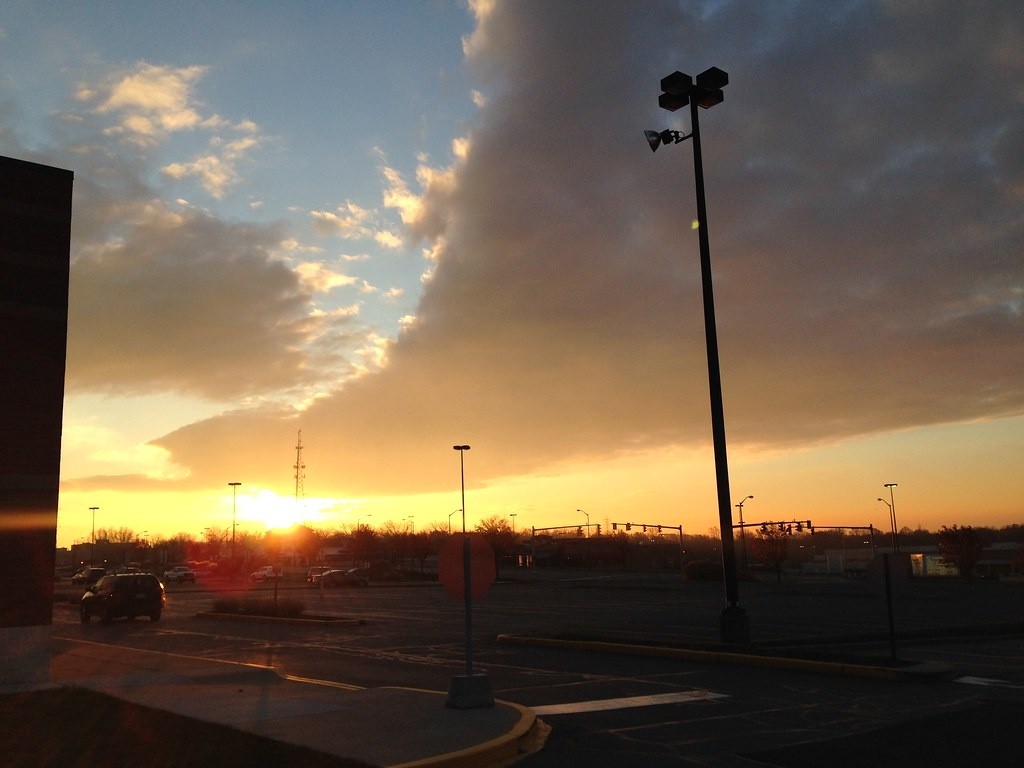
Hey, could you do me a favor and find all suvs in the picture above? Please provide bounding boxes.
[80,569,165,624]
[251,565,283,583]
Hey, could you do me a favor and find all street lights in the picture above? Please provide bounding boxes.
[576,510,589,538]
[228,483,241,580]
[510,514,517,536]
[740,496,754,566]
[453,445,470,532]
[89,507,99,568]
[449,509,463,534]
[658,67,749,646]
[878,483,900,555]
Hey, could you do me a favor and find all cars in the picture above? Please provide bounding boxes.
[306,567,369,588]
[191,568,214,579]
[120,567,142,573]
[72,567,107,586]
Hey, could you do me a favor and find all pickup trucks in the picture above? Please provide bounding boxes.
[164,567,196,585]
